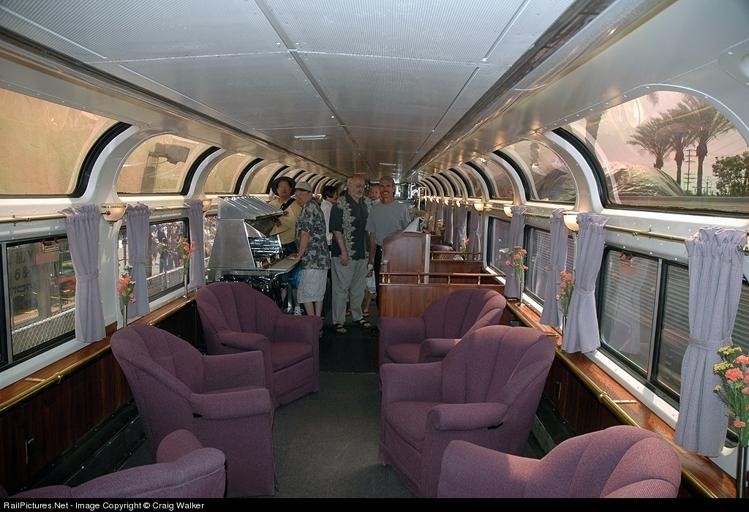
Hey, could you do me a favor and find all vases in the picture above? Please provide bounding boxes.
[561,315,568,343]
[123,304,127,325]
[734,442,745,499]
[182,272,191,298]
[519,279,525,306]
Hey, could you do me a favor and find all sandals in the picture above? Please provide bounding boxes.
[319,326,328,339]
[332,323,348,335]
[352,318,372,328]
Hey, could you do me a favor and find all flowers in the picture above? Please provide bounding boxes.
[555,272,574,322]
[510,242,534,284]
[712,338,748,446]
[177,235,195,277]
[119,269,134,307]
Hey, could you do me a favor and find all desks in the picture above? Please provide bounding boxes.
[207,249,305,312]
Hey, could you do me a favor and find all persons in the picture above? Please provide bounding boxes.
[146,214,219,276]
[267,173,412,339]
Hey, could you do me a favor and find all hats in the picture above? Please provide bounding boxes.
[294,180,312,193]
[271,176,297,197]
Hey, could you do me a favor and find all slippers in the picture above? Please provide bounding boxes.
[362,312,370,318]
[345,310,352,316]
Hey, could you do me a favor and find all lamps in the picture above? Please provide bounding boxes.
[101,203,127,223]
[503,206,514,219]
[473,202,484,215]
[199,197,213,219]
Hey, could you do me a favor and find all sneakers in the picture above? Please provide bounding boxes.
[293,309,304,316]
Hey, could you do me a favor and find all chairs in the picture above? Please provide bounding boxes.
[109,318,283,496]
[195,279,326,407]
[371,320,561,498]
[436,420,687,500]
[7,424,229,498]
[370,281,510,370]
